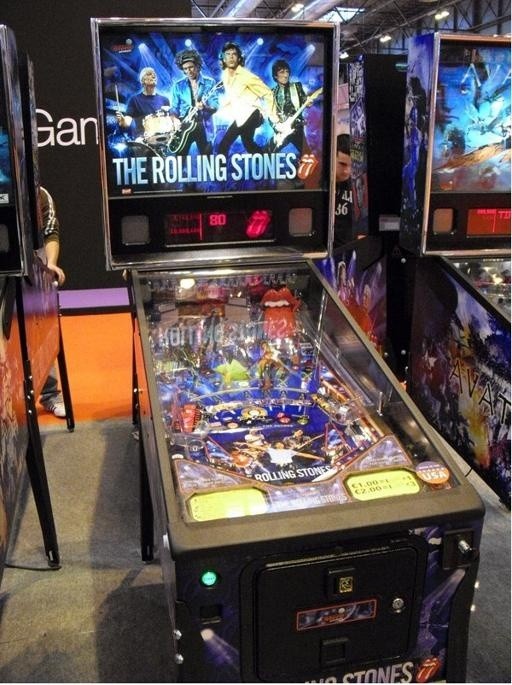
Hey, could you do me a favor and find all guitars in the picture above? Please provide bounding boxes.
[166,80,224,154]
[273,87,324,149]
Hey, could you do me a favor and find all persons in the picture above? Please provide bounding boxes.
[0,134,67,418]
[168,49,220,172]
[333,131,353,251]
[114,67,171,157]
[265,60,314,158]
[215,42,275,158]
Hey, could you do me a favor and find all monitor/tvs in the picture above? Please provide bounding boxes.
[88,18,342,270]
[417,32,511,260]
[0,23,46,280]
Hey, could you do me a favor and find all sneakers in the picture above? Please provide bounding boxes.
[40,395,66,417]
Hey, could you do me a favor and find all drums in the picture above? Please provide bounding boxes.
[143,110,181,146]
